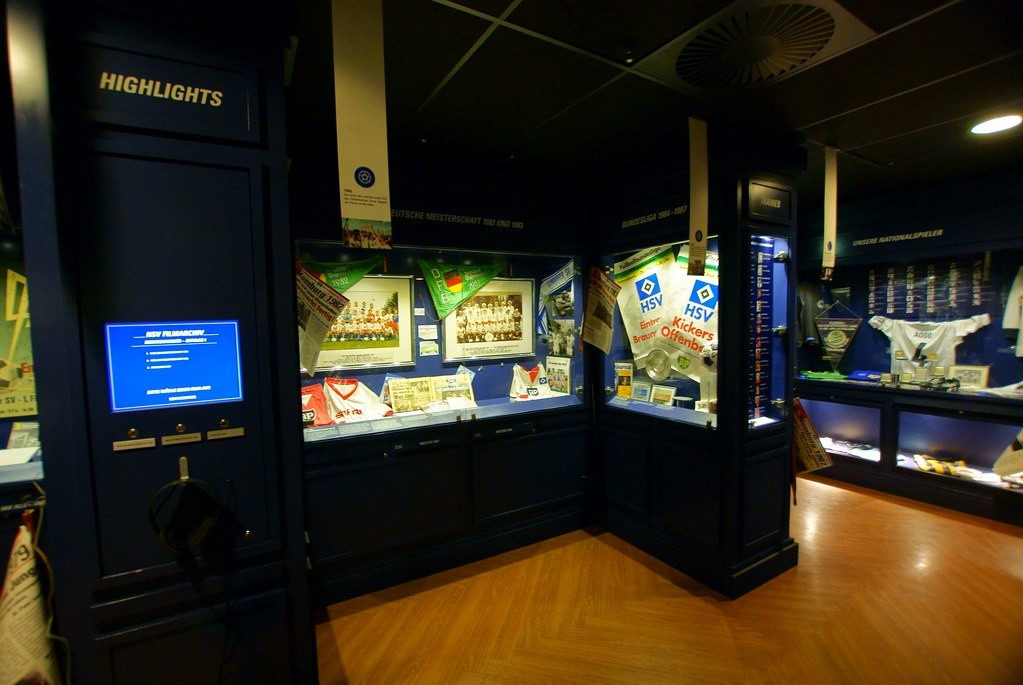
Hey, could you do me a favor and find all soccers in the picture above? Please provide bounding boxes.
[485,333,494,341]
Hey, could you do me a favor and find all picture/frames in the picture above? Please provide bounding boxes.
[442,277,536,364]
[300,274,417,374]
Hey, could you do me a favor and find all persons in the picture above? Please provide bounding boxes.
[326,301,398,341]
[396,403,422,412]
[456,301,523,342]
[619,377,627,385]
[412,381,429,392]
[955,371,978,384]
[553,290,572,315]
[547,368,566,388]
[550,327,575,356]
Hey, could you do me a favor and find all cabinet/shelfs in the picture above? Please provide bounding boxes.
[795,372,1023,526]
[310,399,798,599]
